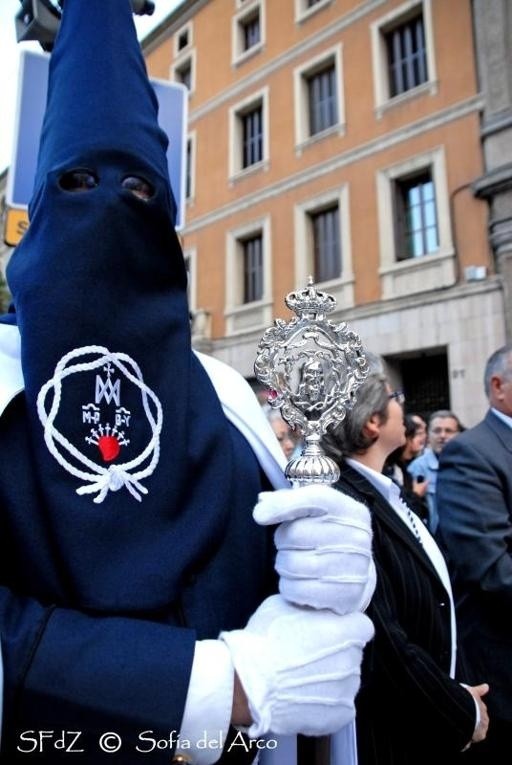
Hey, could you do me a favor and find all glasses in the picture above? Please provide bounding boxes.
[383,390,406,406]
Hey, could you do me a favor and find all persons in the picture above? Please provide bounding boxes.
[295,355,327,403]
[382,413,431,532]
[303,349,494,764]
[260,403,301,461]
[434,342,512,765]
[1,152,382,765]
[405,407,464,537]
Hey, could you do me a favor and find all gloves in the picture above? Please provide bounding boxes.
[222,592,377,737]
[252,486,375,615]
